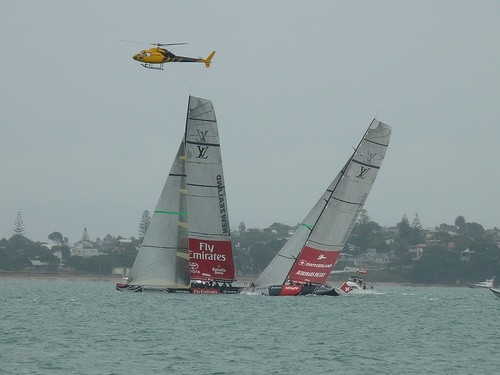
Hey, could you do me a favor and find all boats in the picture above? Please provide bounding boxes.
[466,279,494,288]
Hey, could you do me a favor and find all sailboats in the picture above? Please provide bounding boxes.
[242,119,392,296]
[118,94,245,293]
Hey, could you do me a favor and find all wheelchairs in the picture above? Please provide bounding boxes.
[312,275,369,300]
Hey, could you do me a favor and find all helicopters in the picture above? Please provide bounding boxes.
[131,43,216,71]
